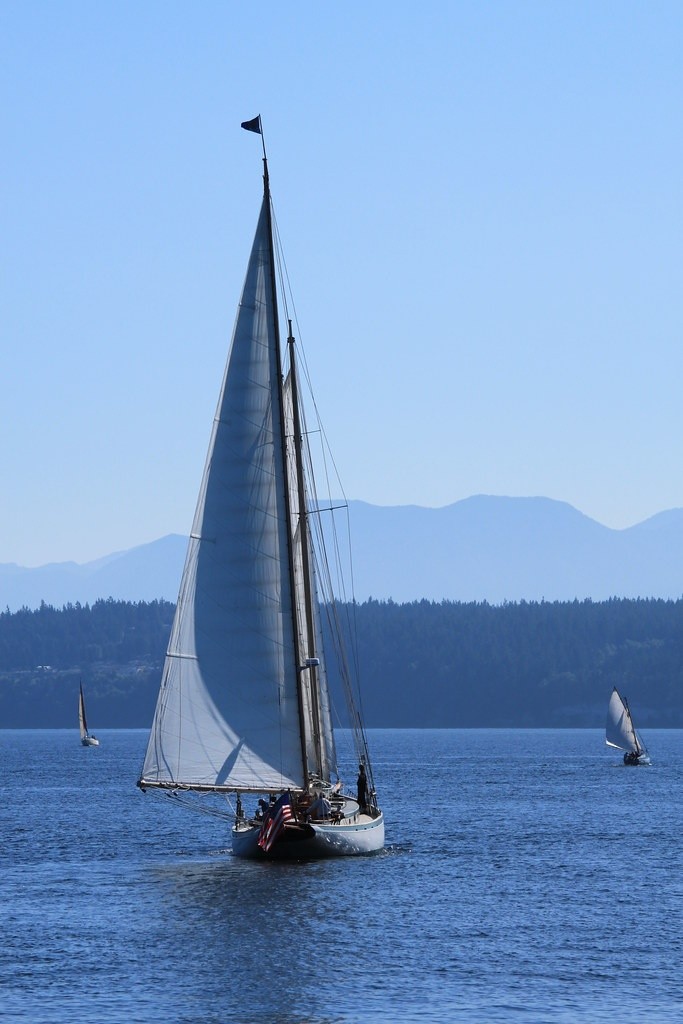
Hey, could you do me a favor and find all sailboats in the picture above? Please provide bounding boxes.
[77,678,100,746]
[135,112,386,859]
[604,685,653,765]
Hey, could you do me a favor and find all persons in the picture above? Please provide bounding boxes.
[303,790,345,822]
[259,799,269,813]
[358,764,368,814]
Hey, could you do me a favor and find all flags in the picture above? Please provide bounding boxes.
[258,790,295,851]
